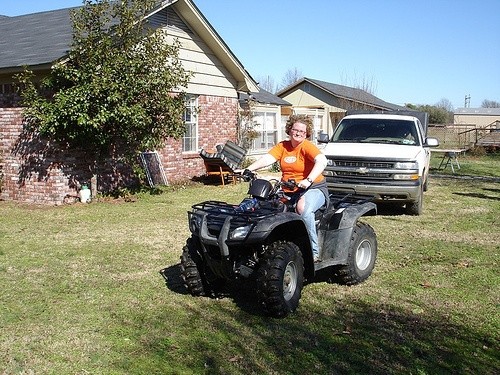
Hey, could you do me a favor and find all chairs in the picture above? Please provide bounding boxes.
[205,150,235,186]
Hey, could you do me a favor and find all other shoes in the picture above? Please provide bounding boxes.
[314,254,319,263]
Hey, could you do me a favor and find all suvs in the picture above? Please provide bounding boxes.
[317,114,440,215]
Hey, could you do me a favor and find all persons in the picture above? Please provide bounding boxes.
[234,114,330,266]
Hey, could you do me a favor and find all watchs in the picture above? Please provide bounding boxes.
[305,178,313,184]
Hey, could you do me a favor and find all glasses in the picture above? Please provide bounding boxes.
[291,129,307,135]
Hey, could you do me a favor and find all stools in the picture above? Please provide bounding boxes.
[227,172,241,184]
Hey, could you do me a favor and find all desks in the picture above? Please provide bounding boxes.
[430,149,466,173]
